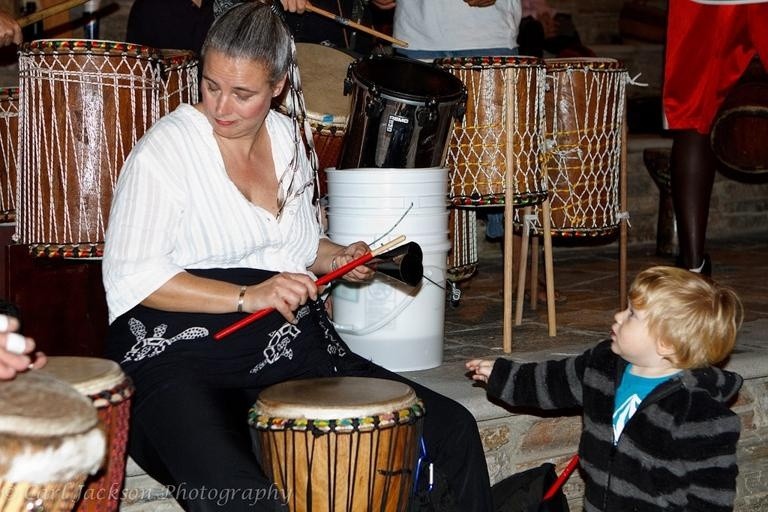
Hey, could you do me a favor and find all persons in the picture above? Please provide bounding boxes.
[465,265,744,512]
[129,0,214,105]
[376,0,523,244]
[0,312,48,380]
[663,0,768,282]
[275,0,387,67]
[0,11,24,48]
[102,3,493,512]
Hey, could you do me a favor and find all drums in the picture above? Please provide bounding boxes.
[709,56,765,183]
[29,357,135,511]
[501,58,629,245]
[0,38,199,259]
[0,369,107,511]
[337,53,468,169]
[248,376,426,512]
[295,42,358,198]
[433,55,547,212]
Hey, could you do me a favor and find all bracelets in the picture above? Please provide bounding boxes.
[238,284,247,314]
[330,257,339,273]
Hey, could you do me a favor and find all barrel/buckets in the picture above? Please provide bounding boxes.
[325,48,468,374]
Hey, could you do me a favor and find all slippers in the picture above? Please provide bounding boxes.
[501,279,568,306]
